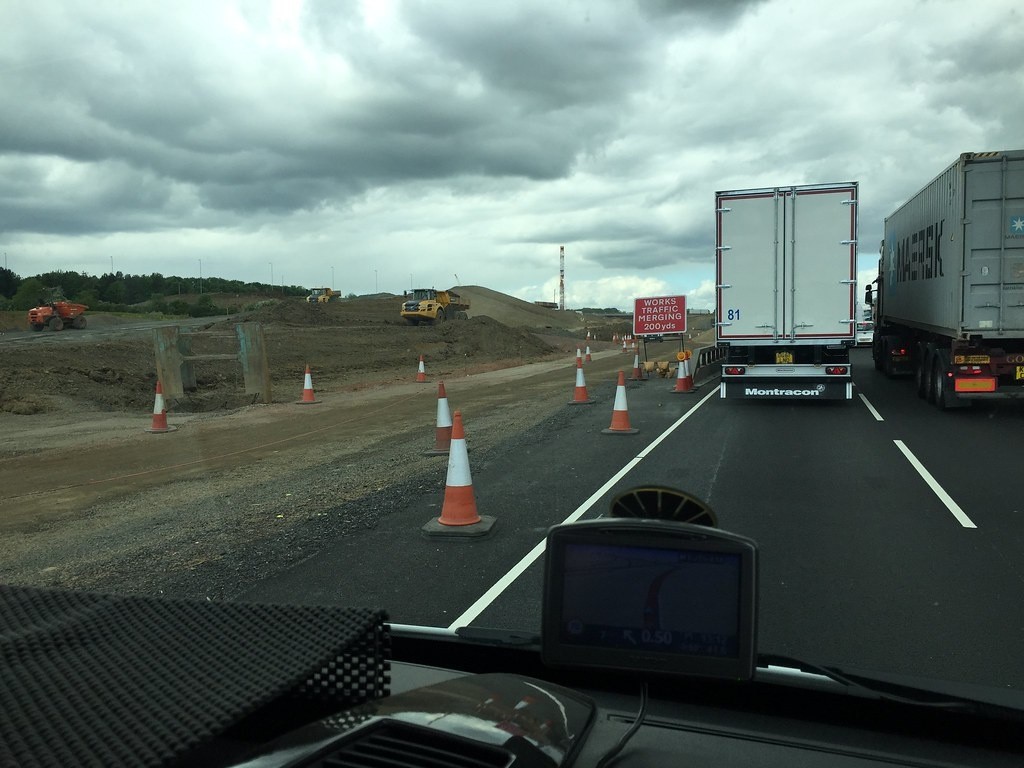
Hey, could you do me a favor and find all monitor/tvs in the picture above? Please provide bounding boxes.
[542,518,760,682]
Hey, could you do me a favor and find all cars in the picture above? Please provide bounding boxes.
[852,321,875,347]
[862,309,872,322]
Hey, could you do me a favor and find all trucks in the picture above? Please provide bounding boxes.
[400,288,469,326]
[306,288,341,303]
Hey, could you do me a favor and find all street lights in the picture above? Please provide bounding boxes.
[198,259,413,294]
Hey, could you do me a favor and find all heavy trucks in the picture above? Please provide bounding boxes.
[713,150,1024,411]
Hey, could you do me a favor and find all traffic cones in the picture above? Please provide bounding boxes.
[586,332,591,344]
[420,411,499,541]
[413,355,431,383]
[144,381,176,433]
[670,352,698,393]
[423,381,471,456]
[600,370,640,435]
[612,332,632,341]
[583,346,593,366]
[293,364,322,404]
[567,345,596,405]
[628,348,648,380]
[620,338,628,354]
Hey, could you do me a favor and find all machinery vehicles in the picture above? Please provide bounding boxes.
[28,301,89,332]
